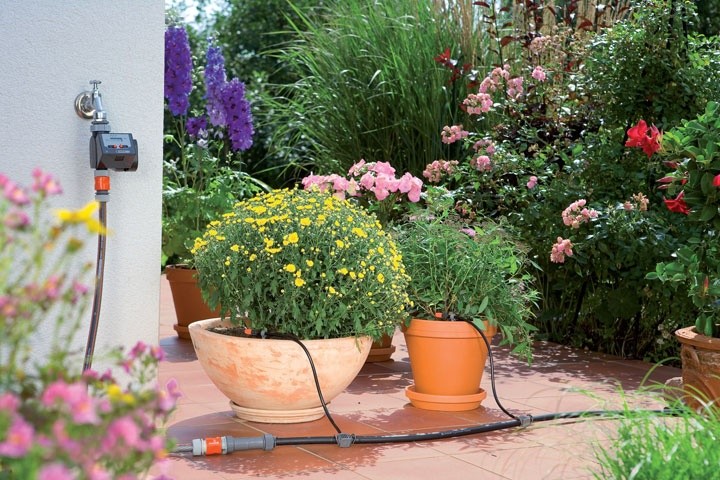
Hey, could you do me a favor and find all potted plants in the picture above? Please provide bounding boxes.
[395,220,542,411]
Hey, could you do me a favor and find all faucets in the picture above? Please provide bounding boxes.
[85,80,109,124]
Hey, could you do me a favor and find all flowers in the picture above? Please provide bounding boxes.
[189,183,414,342]
[625,99,720,337]
[161,21,274,267]
[301,157,425,233]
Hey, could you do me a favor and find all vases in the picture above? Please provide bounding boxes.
[366,326,396,362]
[181,312,371,422]
[673,323,720,410]
[165,264,231,341]
